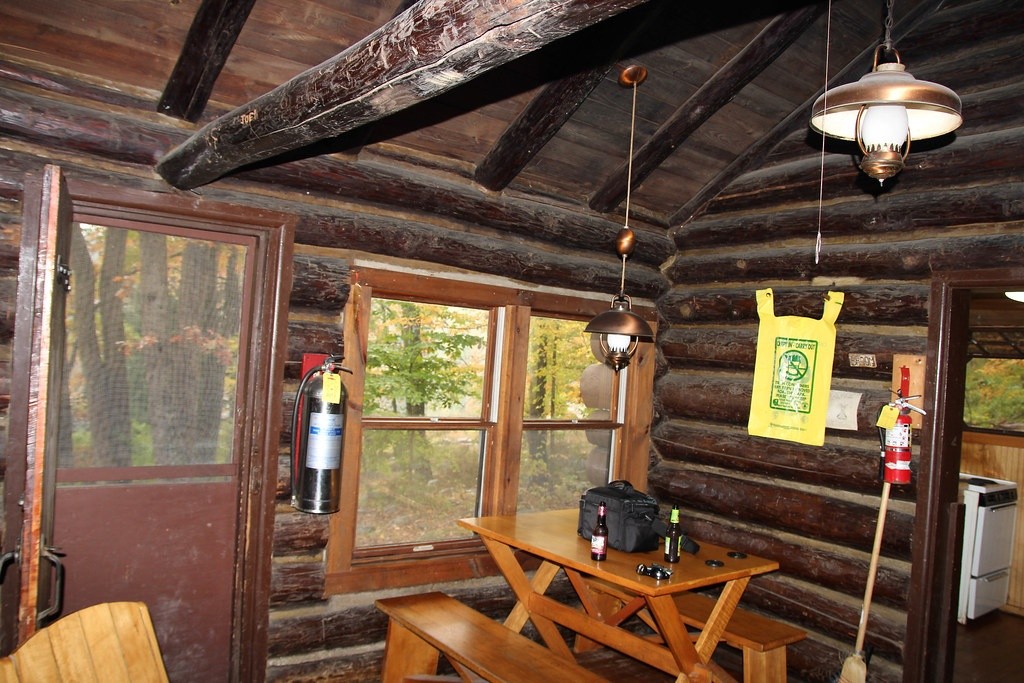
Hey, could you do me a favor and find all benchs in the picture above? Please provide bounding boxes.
[374,592,609,683]
[573,576,805,683]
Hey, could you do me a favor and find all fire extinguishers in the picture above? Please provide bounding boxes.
[876,388,927,486]
[289,354,354,514]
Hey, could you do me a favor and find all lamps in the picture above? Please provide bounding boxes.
[584,65,654,374]
[810,0,962,188]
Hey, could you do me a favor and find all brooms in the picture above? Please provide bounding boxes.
[835,482,891,683]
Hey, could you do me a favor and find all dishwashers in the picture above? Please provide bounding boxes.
[968,489,1017,620]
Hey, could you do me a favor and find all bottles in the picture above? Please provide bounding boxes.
[664,504,681,563]
[591,501,608,562]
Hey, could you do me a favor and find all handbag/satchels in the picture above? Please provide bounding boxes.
[577,479,701,554]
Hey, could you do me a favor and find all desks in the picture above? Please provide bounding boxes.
[458,508,780,683]
